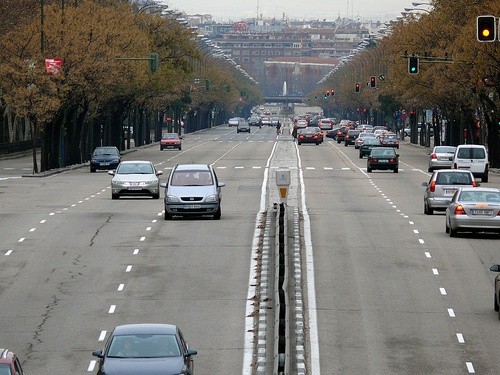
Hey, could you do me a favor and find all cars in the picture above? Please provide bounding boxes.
[443,187,500,238]
[160,133,182,151]
[422,169,481,216]
[108,160,163,201]
[0,348,23,375]
[490,265,500,320]
[93,323,197,375]
[89,146,122,173]
[449,145,489,182]
[290,112,456,173]
[160,164,225,220]
[228,105,282,134]
[123,126,133,140]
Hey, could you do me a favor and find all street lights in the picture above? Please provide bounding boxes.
[350,35,387,83]
[189,38,220,73]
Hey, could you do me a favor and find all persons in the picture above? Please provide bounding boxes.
[306,116,310,127]
[292,125,300,141]
[276,121,281,135]
[259,119,262,129]
[294,119,298,126]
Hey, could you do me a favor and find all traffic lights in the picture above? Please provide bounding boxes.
[356,84,360,93]
[408,54,419,74]
[308,89,334,102]
[371,76,376,88]
[477,16,496,42]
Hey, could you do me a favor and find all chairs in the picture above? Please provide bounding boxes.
[462,193,499,201]
[122,165,147,170]
[439,175,468,182]
[177,173,210,181]
[374,151,391,154]
